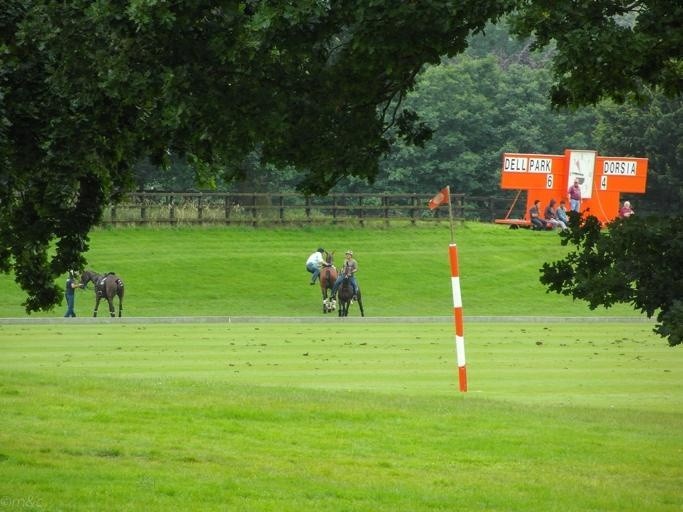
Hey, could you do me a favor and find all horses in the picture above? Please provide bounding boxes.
[78,269,127,317]
[337,264,365,317]
[318,249,338,314]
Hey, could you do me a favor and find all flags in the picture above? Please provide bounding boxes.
[425,186,448,213]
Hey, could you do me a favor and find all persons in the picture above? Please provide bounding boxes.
[304,248,330,285]
[619,200,635,219]
[62,274,84,317]
[554,200,568,225]
[528,200,547,231]
[544,199,567,232]
[566,179,582,212]
[328,250,359,303]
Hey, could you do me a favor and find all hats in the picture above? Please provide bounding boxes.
[346,250,353,256]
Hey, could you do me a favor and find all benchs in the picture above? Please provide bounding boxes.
[495,218,552,230]
